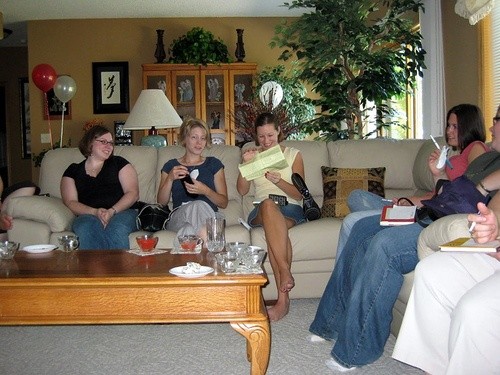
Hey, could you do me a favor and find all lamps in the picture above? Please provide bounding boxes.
[124,89,184,148]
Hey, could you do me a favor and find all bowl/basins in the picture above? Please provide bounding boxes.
[177,235,198,250]
[0,240,19,260]
[56,234,79,252]
[136,235,158,252]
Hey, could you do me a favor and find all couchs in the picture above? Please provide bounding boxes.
[2,139,473,336]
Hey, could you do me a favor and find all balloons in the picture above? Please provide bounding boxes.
[53,75,77,102]
[32,63,55,92]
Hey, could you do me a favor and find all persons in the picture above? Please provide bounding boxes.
[308,102,492,370]
[236,113,305,323]
[157,118,228,248]
[392,104,500,375]
[61,125,140,251]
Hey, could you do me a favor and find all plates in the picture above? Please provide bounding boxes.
[23,244,59,253]
[211,137,225,145]
[168,265,215,278]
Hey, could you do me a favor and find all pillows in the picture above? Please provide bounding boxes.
[320,163,389,222]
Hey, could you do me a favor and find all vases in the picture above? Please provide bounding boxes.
[155,29,164,62]
[234,29,245,62]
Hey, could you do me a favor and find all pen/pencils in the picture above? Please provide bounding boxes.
[430,134,454,169]
[468,195,492,233]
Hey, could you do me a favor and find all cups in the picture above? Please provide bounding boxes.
[216,242,266,273]
[206,213,225,252]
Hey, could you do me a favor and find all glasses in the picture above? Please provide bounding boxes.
[96,139,115,146]
[493,116,500,126]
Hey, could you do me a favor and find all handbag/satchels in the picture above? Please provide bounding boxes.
[138,201,170,232]
[292,172,321,221]
[414,175,487,228]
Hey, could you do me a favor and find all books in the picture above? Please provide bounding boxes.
[438,237,500,252]
[380,203,414,226]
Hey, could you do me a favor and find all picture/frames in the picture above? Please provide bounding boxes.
[43,75,71,119]
[90,62,131,113]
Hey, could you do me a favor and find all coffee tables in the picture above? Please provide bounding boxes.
[0,248,271,375]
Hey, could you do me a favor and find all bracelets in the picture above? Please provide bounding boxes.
[111,207,116,215]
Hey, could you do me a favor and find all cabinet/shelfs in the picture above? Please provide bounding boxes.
[141,60,258,147]
